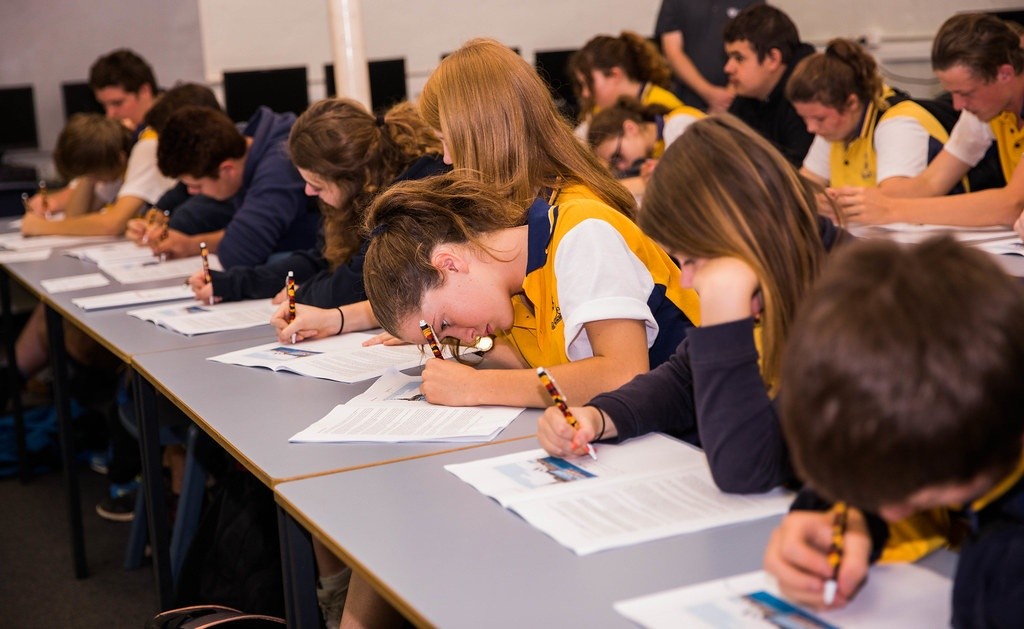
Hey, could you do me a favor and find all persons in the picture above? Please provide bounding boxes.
[0,0,1024,629]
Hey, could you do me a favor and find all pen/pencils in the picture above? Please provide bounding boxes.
[286,270,299,345]
[537,366,600,464]
[142,207,157,243]
[40,181,52,220]
[419,318,445,361]
[824,498,847,607]
[160,210,169,262]
[200,242,215,306]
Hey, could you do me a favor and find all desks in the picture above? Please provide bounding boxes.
[0,217,954,628]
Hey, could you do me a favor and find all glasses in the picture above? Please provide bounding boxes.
[608,132,626,171]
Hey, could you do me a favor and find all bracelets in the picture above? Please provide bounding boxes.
[337,308,344,334]
[594,407,606,442]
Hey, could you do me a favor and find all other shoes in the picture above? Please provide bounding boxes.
[95,452,215,522]
[315,580,349,629]
[118,401,191,438]
